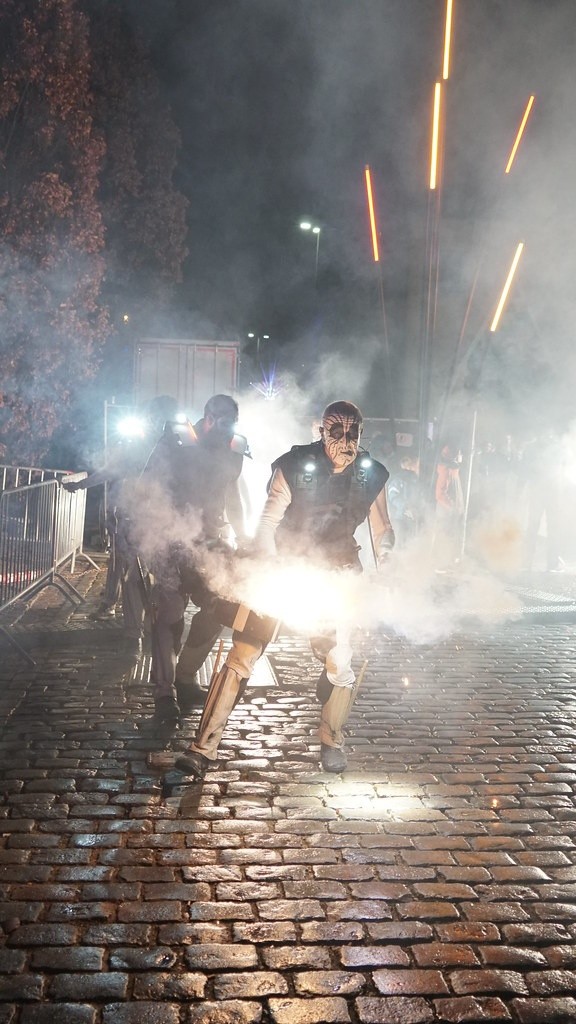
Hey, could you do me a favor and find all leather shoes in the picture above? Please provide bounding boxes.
[322,742,347,772]
[175,750,209,779]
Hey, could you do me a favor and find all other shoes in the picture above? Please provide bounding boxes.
[174,680,209,701]
[155,696,182,719]
[124,636,142,663]
[88,608,117,621]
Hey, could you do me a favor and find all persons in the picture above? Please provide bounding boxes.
[311,419,576,574]
[174,401,395,779]
[63,394,252,724]
